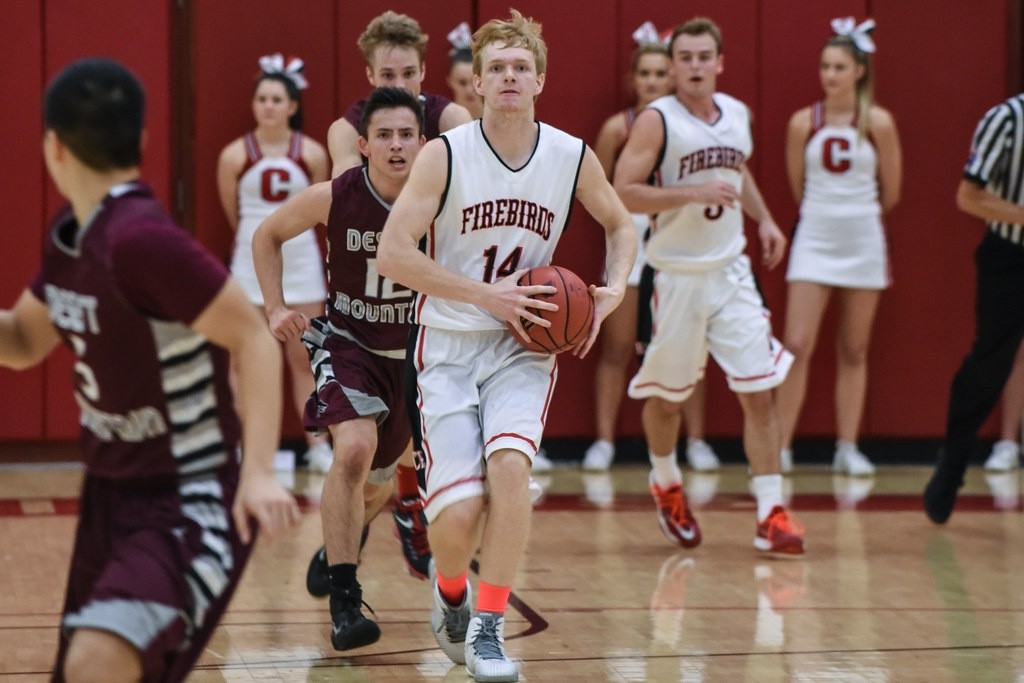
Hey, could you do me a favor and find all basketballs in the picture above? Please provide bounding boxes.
[506,266,595,355]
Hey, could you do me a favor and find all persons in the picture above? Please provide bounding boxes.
[375,8,639,683]
[445,23,483,119]
[252,87,426,652]
[922,92,1024,525]
[985,340,1024,471]
[613,16,806,560]
[327,11,474,581]
[216,53,334,473]
[0,59,302,683]
[583,21,720,471]
[773,17,901,476]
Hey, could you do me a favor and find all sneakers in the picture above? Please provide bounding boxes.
[390,500,429,582]
[646,469,703,548]
[752,506,806,558]
[983,442,1019,471]
[304,449,333,475]
[427,557,475,664]
[582,442,615,471]
[832,445,877,475]
[688,441,721,472]
[536,455,555,472]
[328,586,382,651]
[306,528,369,600]
[464,613,518,683]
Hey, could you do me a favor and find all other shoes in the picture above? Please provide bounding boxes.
[922,468,964,525]
